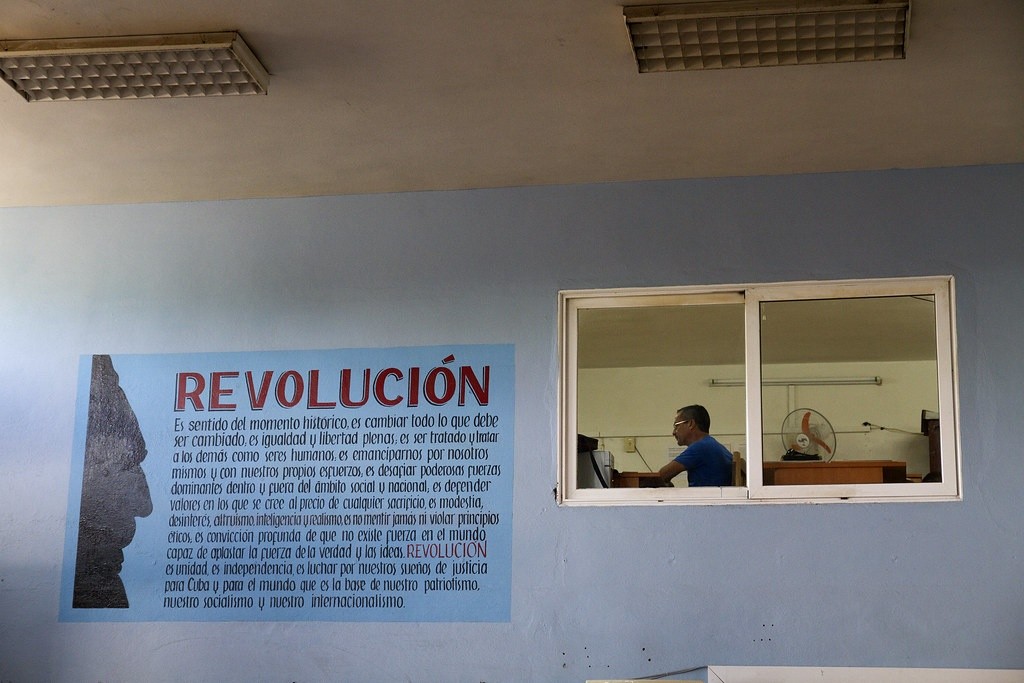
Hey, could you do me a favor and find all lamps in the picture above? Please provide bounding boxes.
[709,375,881,386]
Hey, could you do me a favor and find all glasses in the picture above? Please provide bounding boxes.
[673,419,689,427]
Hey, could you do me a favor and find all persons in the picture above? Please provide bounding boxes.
[659,405,733,487]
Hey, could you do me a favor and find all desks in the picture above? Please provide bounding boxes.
[762,460,907,485]
[613,472,662,488]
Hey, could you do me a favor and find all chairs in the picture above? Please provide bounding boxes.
[733,451,747,486]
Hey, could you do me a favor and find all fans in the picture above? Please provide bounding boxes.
[781,408,838,462]
